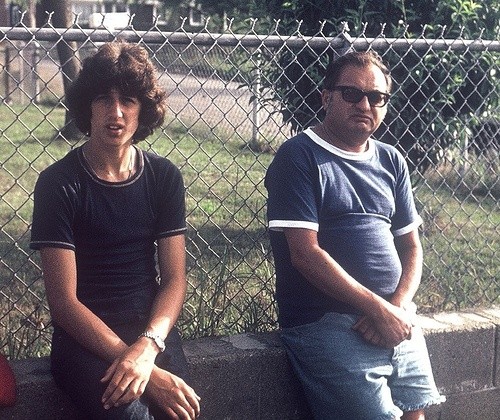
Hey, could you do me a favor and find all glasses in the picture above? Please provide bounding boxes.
[330,86,392,108]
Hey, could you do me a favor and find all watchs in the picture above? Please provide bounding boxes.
[137,332,166,352]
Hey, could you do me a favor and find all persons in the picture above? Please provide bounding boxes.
[263,50,447,420]
[31,40,203,420]
